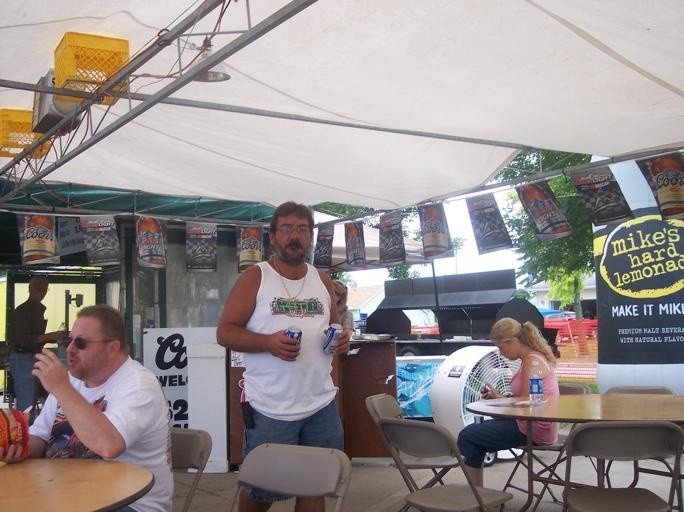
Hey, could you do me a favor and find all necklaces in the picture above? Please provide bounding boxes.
[273,255,307,299]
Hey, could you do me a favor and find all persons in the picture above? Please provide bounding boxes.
[216,203,355,511]
[330,278,353,329]
[457,318,560,488]
[0,304,172,512]
[8,276,65,426]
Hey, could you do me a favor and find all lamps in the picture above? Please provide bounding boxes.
[188,34,232,83]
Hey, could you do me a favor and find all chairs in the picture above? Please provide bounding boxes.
[602,384,682,508]
[502,384,594,512]
[170,429,212,511]
[560,420,683,511]
[366,392,465,511]
[229,443,352,512]
[377,415,515,510]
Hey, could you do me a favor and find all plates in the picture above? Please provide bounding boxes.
[351,333,392,340]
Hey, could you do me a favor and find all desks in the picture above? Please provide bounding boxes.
[464,394,683,488]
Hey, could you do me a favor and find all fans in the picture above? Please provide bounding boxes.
[430,345,523,467]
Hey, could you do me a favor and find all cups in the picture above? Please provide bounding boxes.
[359,312,367,320]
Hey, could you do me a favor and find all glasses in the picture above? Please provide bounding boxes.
[64,337,114,350]
[276,225,312,235]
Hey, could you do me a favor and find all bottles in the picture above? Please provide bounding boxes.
[55,321,67,353]
[517,184,570,240]
[343,222,366,269]
[21,215,56,263]
[238,225,263,271]
[528,360,543,405]
[420,204,448,258]
[653,153,683,218]
[416,331,422,341]
[134,215,165,268]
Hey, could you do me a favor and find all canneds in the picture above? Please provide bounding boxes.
[286,328,302,351]
[322,323,345,355]
